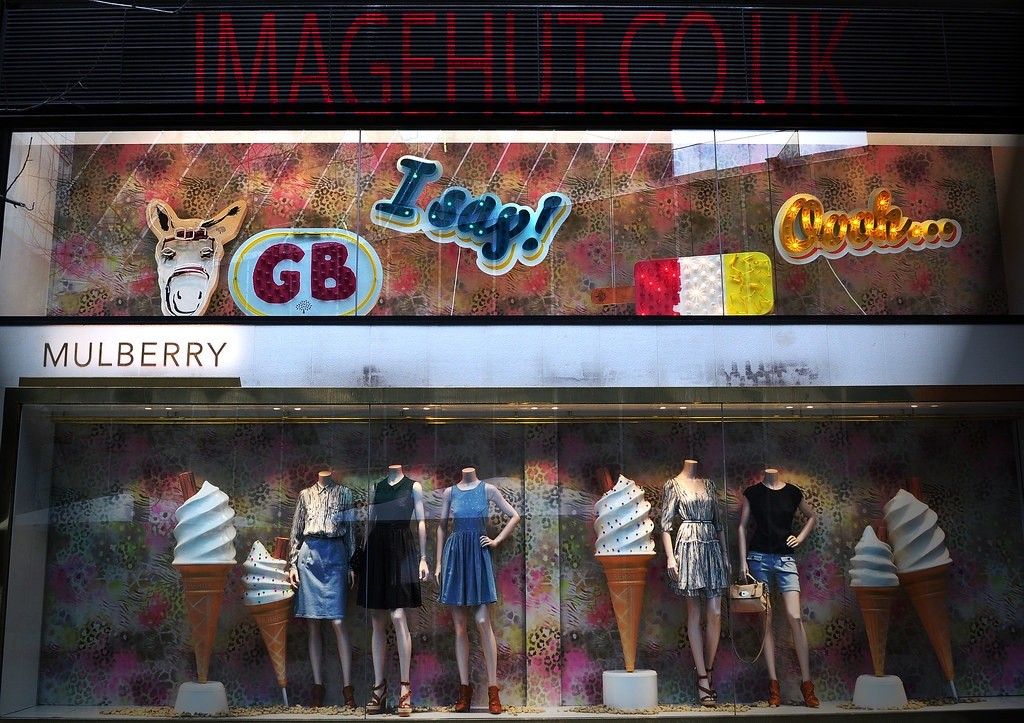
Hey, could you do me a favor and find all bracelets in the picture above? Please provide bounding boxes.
[420,556,426,559]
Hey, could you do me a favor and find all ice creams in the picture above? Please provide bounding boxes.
[173,479,296,711]
[851,487,961,700]
[592,475,656,672]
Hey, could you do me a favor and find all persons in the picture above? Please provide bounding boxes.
[737,469,820,708]
[288,471,356,712]
[661,460,732,705]
[350,464,429,716]
[432,468,520,713]
